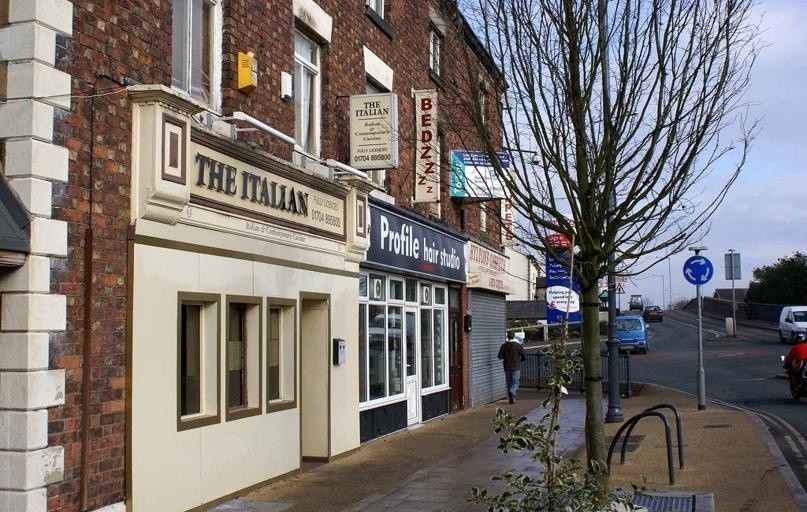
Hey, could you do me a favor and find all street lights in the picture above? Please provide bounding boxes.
[652,274,664,309]
[656,256,671,310]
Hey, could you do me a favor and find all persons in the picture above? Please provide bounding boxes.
[498,331,527,404]
[782,333,807,392]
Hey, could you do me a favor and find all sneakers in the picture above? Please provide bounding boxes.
[508,393,514,404]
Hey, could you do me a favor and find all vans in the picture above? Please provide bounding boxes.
[614,315,650,355]
[368,311,416,348]
[776,304,806,344]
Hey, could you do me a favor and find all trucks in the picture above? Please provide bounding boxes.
[627,294,643,311]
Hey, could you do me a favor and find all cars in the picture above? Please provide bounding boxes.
[642,305,663,321]
[779,340,806,400]
[505,332,523,347]
